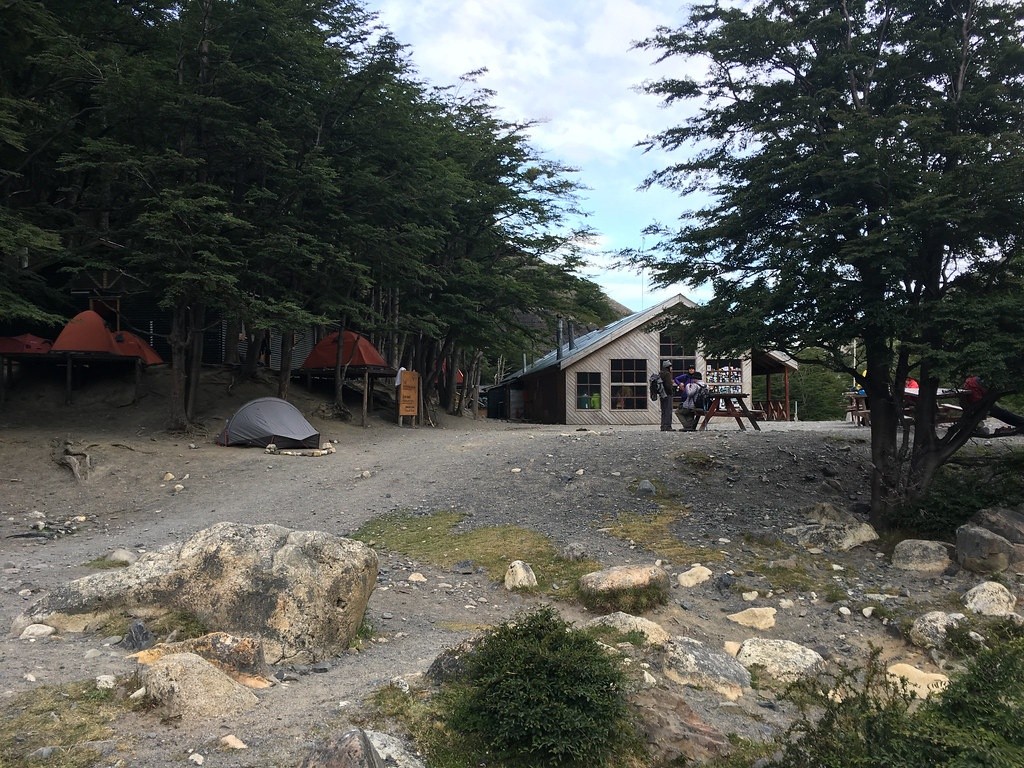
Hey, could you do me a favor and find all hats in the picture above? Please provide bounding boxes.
[690,372,702,380]
[688,364,695,369]
[663,360,672,368]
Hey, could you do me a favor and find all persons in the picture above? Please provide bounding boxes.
[856,369,871,426]
[590,390,601,409]
[905,378,919,388]
[959,375,988,420]
[659,360,707,431]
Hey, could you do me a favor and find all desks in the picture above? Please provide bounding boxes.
[847,393,870,427]
[699,393,761,431]
[904,387,971,426]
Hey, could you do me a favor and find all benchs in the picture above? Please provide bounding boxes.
[845,403,988,423]
[690,409,766,419]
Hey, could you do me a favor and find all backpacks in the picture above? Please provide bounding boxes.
[693,383,711,410]
[649,371,670,394]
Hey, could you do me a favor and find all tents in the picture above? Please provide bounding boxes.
[301,330,388,369]
[0,310,163,366]
[213,397,320,449]
[456,370,463,385]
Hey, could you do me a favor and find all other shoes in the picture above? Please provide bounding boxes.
[679,427,694,432]
[663,426,675,431]
